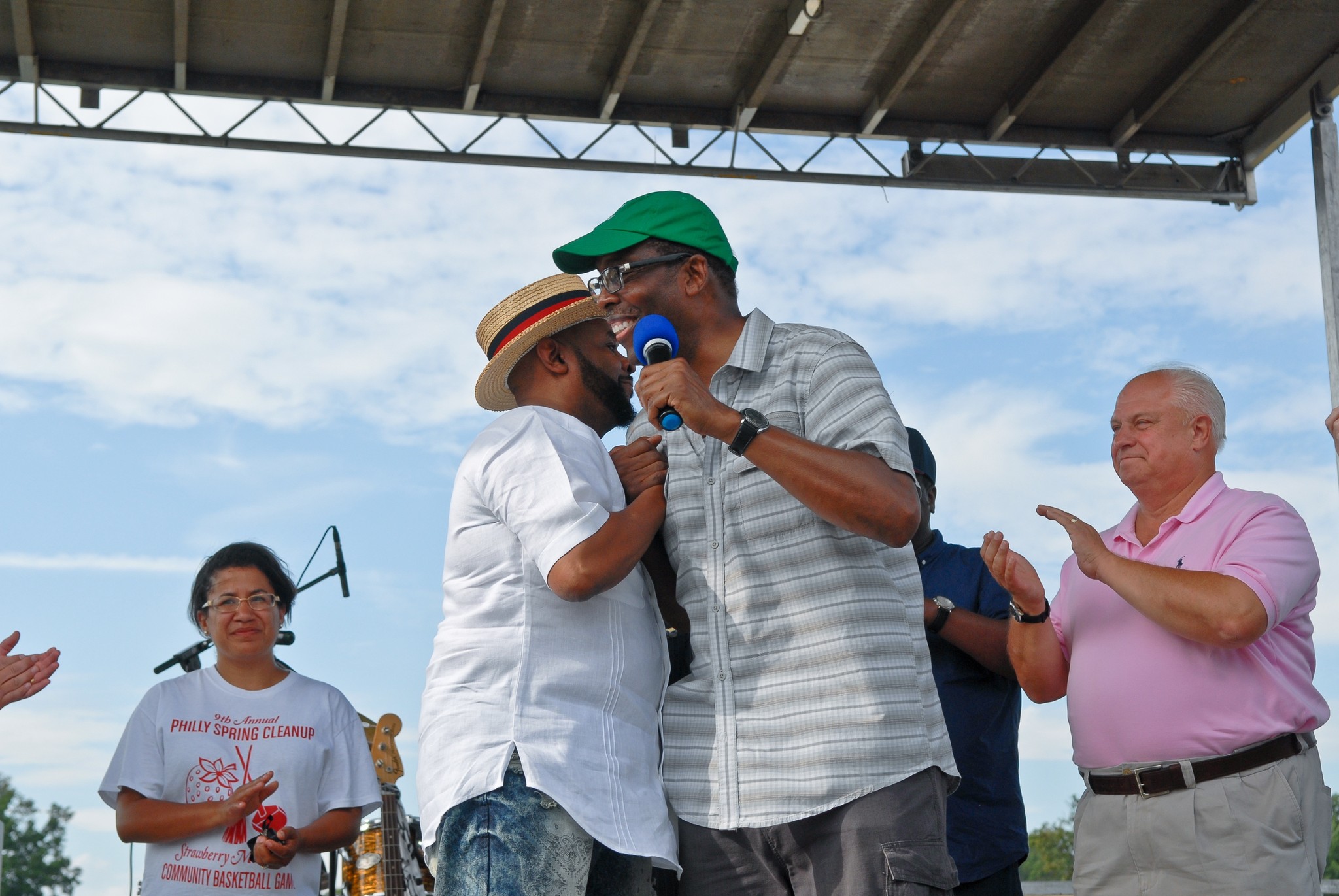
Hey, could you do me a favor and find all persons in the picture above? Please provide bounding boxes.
[905,426,1029,896]
[98,543,383,896]
[417,273,682,896]
[980,367,1332,896]
[0,631,60,710]
[552,191,963,896]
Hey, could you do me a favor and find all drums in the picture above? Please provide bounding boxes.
[348,815,425,896]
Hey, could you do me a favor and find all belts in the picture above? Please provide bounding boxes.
[1082,730,1318,796]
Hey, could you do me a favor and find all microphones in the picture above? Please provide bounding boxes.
[333,525,350,598]
[632,314,683,432]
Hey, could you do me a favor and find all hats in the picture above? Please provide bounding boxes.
[905,427,936,484]
[552,191,738,274]
[475,274,612,411]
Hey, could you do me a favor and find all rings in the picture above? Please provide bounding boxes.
[31,678,34,685]
[1071,517,1078,523]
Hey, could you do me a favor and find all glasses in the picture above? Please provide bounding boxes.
[202,592,281,613]
[587,252,715,299]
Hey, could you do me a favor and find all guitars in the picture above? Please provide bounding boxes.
[368,714,408,896]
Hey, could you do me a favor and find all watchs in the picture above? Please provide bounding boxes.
[929,596,954,634]
[1010,596,1051,624]
[728,408,769,457]
